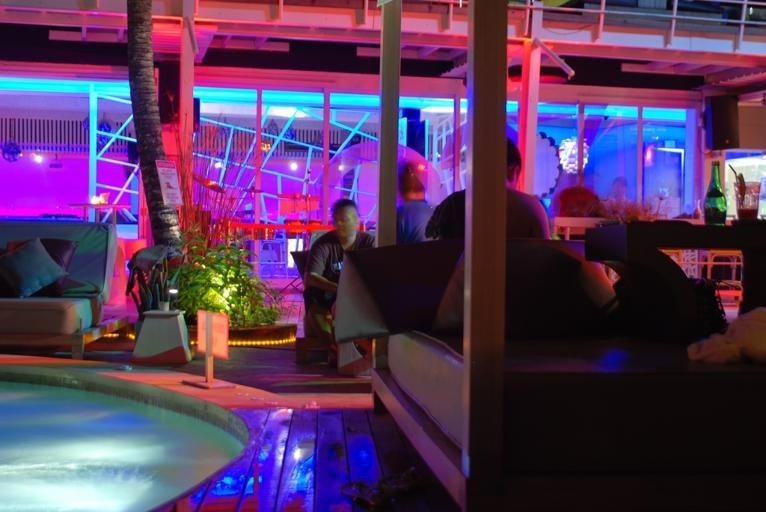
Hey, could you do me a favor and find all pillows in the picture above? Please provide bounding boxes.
[432,241,620,340]
[0,236,78,299]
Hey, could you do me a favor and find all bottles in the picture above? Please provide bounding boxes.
[703,158,727,228]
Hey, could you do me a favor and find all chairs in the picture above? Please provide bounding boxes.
[289,251,327,371]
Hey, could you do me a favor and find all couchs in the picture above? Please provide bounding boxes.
[0,220,116,334]
[331,236,766,475]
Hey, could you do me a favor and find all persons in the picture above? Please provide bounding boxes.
[601,175,635,214]
[555,173,611,216]
[425,136,549,242]
[396,161,439,246]
[302,201,377,368]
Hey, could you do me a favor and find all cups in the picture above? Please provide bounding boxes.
[733,180,762,222]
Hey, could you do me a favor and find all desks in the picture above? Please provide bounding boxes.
[584,220,766,337]
